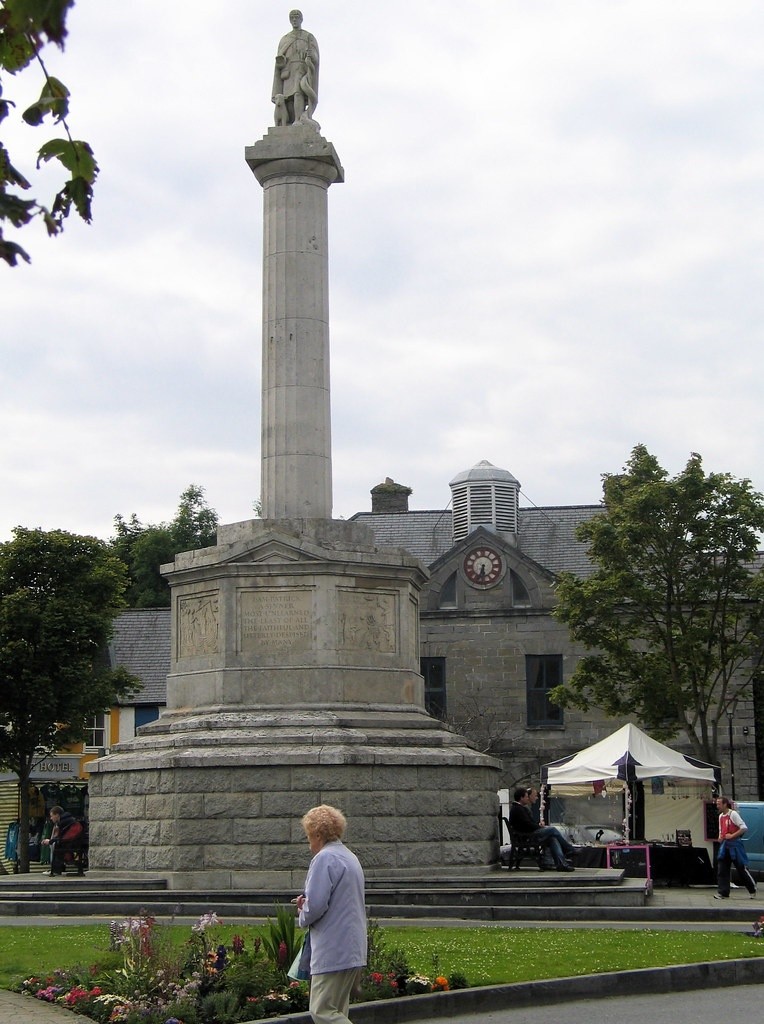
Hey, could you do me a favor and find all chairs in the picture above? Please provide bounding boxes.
[52,818,87,878]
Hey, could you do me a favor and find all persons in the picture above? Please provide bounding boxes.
[508,789,584,873]
[527,787,558,870]
[713,797,757,900]
[40,805,83,875]
[271,11,319,124]
[297,803,368,1024]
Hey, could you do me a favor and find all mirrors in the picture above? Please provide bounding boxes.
[606,845,650,878]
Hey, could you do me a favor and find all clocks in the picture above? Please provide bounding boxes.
[458,543,506,590]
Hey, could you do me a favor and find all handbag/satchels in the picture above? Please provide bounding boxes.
[286,927,312,981]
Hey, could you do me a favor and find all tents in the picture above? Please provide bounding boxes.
[539,723,715,784]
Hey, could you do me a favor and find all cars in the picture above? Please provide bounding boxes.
[550,824,626,845]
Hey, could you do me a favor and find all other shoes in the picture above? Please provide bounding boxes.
[558,863,576,872]
[41,870,59,876]
[567,848,583,859]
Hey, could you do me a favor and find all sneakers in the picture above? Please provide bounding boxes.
[749,884,757,899]
[714,893,729,900]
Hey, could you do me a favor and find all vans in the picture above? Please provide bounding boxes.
[733,801,764,883]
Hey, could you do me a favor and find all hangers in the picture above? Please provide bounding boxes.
[10,781,89,827]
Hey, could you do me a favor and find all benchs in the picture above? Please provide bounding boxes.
[501,816,548,872]
